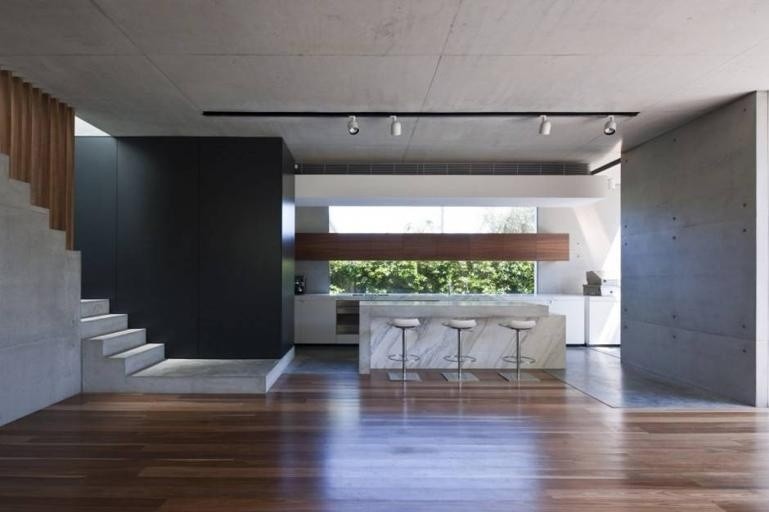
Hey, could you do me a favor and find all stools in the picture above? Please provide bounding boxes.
[388,317,541,384]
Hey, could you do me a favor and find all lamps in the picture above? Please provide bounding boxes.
[536,110,619,140]
[346,110,404,139]
